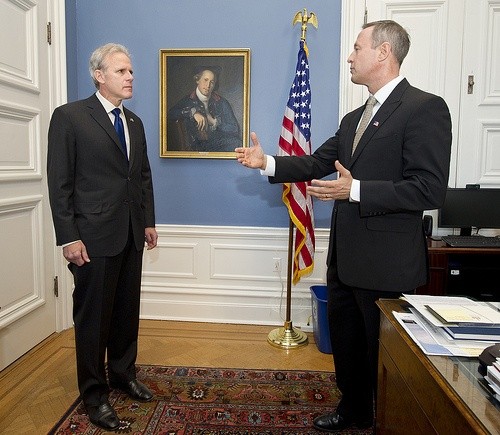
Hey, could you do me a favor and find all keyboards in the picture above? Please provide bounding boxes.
[442,235,500,247]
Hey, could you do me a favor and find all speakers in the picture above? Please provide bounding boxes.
[423,215,433,239]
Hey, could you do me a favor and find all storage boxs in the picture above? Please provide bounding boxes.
[310,286,332,353]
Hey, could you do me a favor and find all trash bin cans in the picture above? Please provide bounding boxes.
[310,285,334,354]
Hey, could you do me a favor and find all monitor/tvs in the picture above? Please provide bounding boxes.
[438,188,500,236]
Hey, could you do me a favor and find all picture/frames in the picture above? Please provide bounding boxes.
[159,48,251,158]
[272,258,281,272]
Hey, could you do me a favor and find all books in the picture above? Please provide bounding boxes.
[392,294,500,401]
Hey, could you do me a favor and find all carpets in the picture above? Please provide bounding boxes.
[47,362,374,435]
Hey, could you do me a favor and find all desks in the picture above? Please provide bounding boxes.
[427,236,500,295]
[376,296,500,435]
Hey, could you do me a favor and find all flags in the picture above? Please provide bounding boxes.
[277,40,316,285]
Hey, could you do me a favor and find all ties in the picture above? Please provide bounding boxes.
[351,95,378,158]
[111,108,128,161]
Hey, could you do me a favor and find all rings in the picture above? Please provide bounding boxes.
[326,194,327,198]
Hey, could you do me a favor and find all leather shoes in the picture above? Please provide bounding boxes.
[86,400,120,431]
[312,410,373,432]
[124,378,153,403]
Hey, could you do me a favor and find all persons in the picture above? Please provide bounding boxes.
[46,42,158,429]
[234,20,452,431]
